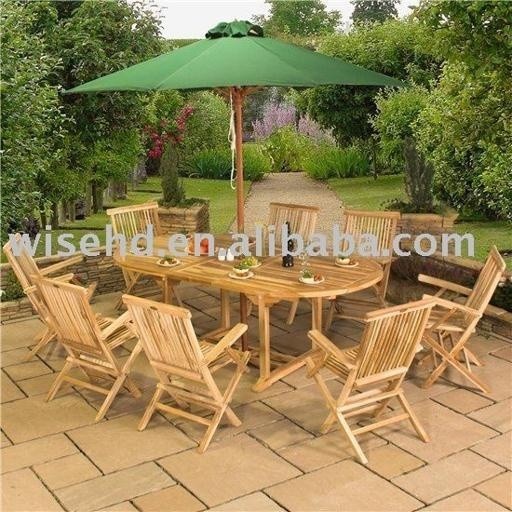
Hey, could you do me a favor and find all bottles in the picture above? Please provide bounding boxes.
[217,248,235,261]
[282,221,293,266]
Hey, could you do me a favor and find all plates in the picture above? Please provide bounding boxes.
[157,256,179,267]
[300,276,324,284]
[228,270,254,279]
[185,247,218,256]
[334,260,359,269]
[241,259,262,268]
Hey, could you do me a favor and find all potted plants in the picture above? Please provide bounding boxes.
[154,141,211,234]
[374,83,479,235]
